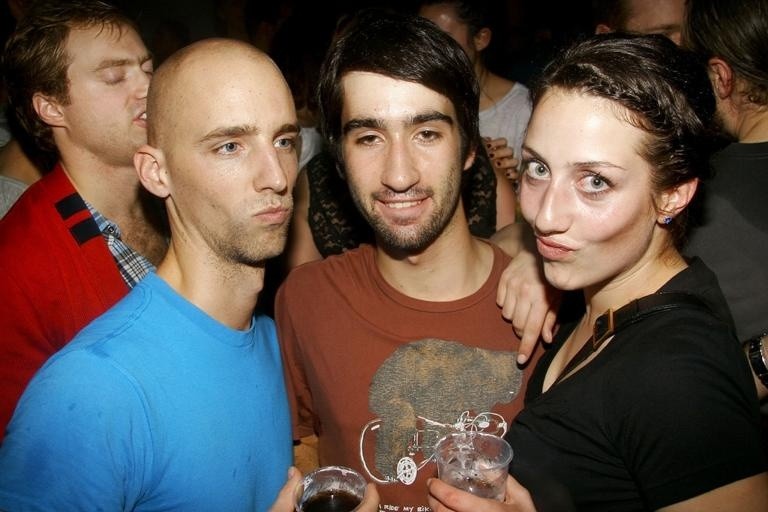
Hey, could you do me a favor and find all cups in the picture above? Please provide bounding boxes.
[434,430,516,502]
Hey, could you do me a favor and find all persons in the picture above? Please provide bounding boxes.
[2,3,768,512]
